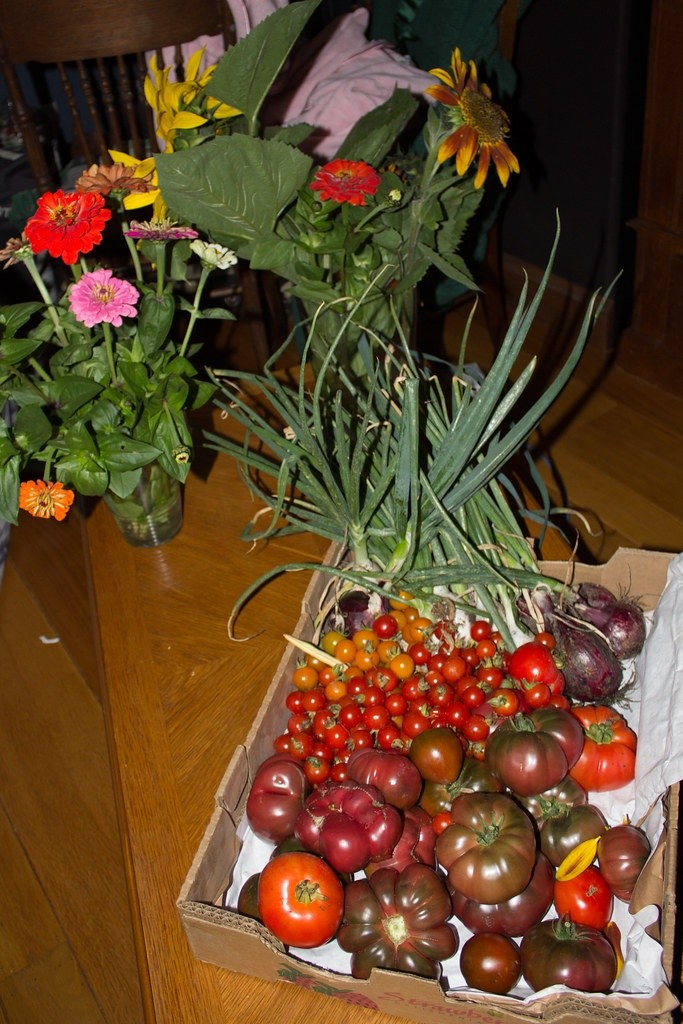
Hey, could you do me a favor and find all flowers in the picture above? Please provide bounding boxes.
[152,0,519,340]
[0,1,318,551]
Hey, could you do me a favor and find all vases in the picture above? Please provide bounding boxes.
[312,326,423,425]
[104,462,186,547]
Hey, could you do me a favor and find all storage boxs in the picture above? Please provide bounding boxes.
[173,524,682,1024]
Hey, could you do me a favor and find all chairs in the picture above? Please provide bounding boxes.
[0,0,289,376]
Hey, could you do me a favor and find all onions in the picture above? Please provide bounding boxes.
[328,583,642,700]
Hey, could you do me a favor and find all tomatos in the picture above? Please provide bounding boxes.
[238,612,650,993]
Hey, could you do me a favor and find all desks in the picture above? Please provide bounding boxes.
[77,332,683,1024]
